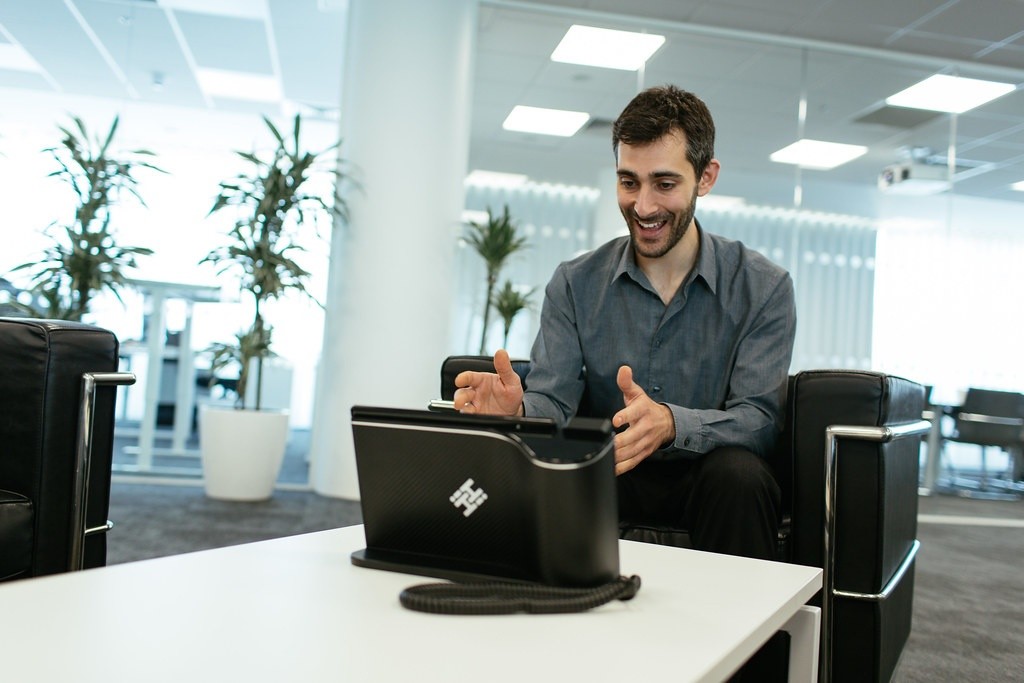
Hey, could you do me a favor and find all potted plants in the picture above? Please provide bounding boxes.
[191,108,345,506]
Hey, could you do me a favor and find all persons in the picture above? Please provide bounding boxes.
[446,81,799,566]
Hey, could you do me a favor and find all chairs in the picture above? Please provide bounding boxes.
[940,388,1024,501]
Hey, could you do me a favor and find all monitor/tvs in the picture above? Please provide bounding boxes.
[352,404,621,578]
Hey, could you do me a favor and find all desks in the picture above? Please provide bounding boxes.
[0,523,830,681]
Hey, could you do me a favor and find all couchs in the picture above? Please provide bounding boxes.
[0,314,136,587]
[438,356,930,682]
